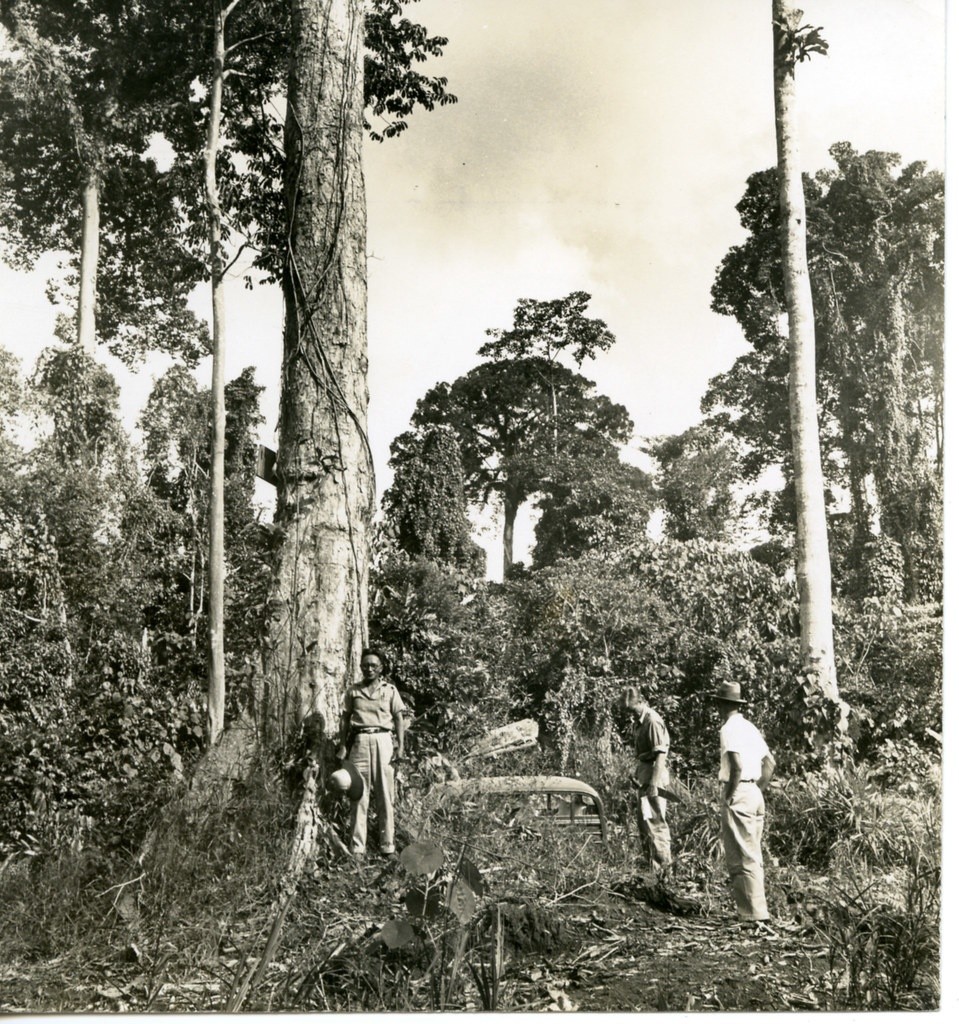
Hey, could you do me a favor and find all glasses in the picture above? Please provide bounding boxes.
[362,663,382,668]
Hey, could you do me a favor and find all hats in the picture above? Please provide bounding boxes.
[709,680,748,703]
[327,760,364,802]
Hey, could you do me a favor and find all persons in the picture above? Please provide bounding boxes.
[708,679,776,929]
[619,686,673,869]
[334,648,406,854]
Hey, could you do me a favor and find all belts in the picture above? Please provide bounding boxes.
[358,728,390,734]
[724,780,754,784]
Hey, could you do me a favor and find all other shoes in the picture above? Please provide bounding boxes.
[353,851,364,862]
[384,853,397,861]
